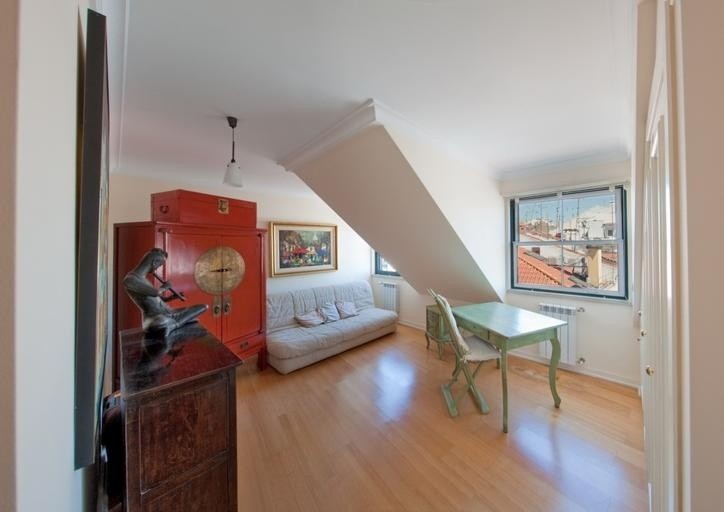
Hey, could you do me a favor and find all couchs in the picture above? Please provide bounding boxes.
[264,280,399,376]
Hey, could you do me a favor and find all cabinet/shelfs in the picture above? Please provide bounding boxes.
[109,220,268,387]
[627,1,724,512]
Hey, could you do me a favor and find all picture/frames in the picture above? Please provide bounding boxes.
[74,7,114,473]
[268,221,340,278]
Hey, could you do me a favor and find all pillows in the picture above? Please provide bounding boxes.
[319,300,341,323]
[294,306,324,328]
[336,302,358,319]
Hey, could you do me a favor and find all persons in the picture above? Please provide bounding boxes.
[121,247,208,337]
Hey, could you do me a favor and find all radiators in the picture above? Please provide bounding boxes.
[381,282,401,314]
[537,303,578,367]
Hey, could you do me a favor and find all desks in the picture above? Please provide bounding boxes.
[424,304,454,357]
[448,301,568,433]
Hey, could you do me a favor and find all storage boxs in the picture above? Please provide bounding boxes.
[149,189,258,229]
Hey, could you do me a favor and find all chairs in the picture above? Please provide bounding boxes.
[425,287,502,417]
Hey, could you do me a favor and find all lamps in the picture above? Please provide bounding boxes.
[221,116,243,187]
